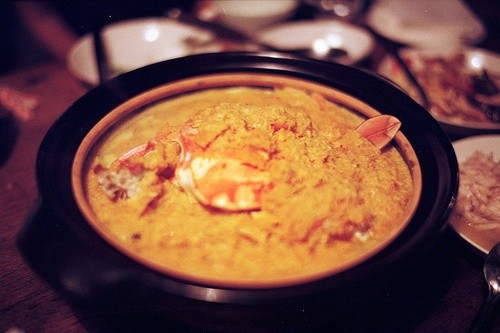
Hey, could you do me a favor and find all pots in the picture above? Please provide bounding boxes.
[36,52,460,319]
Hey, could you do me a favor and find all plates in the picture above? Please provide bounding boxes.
[249,19,373,64]
[70,19,224,84]
[380,44,500,129]
[451,135,500,253]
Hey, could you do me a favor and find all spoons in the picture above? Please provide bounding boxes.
[182,14,348,59]
[466,242,500,333]
